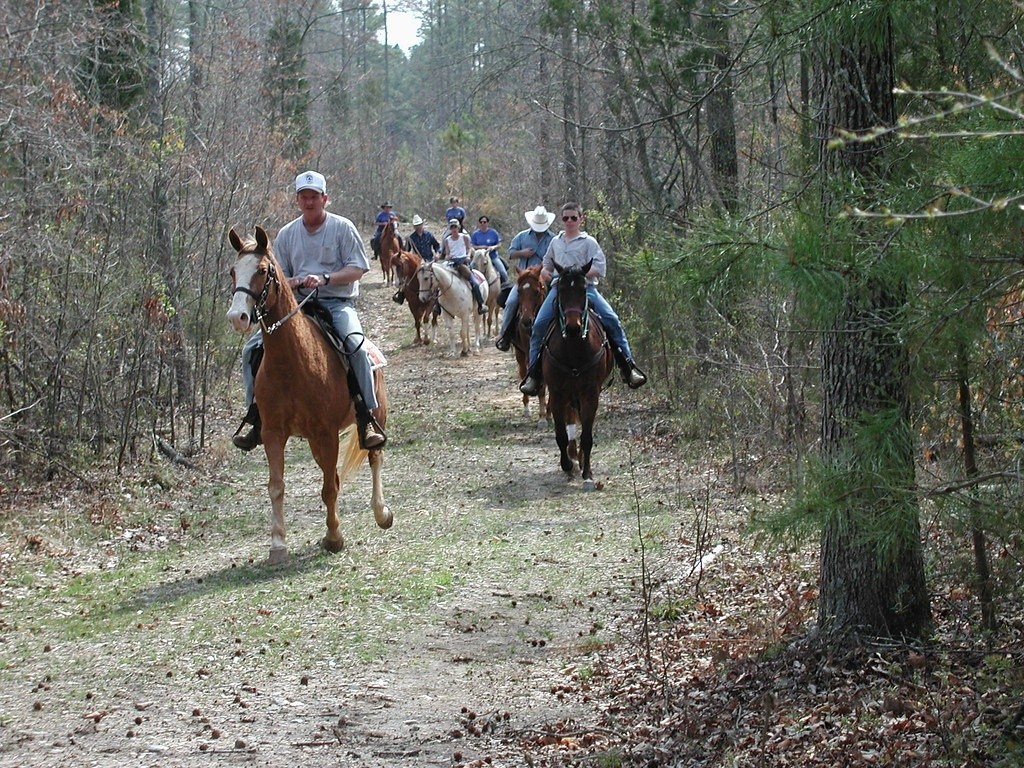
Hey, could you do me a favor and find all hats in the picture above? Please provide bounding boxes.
[412,214,423,225]
[478,215,490,222]
[295,171,326,196]
[450,197,459,203]
[525,205,556,232]
[380,201,393,208]
[449,219,461,229]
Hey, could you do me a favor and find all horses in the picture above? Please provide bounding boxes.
[226,225,393,566]
[379,215,615,491]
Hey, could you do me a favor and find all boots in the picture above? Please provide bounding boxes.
[392,289,405,304]
[355,414,384,449]
[472,285,489,314]
[234,423,264,449]
[497,319,516,352]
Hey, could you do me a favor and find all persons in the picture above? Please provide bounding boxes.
[496,206,557,350]
[521,202,644,393]
[371,201,397,260]
[233,170,385,448]
[392,215,440,305]
[439,197,489,315]
[471,216,510,284]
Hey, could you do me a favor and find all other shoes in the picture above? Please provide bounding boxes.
[627,363,645,385]
[371,255,378,261]
[521,375,539,394]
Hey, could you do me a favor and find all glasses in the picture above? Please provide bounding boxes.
[562,216,578,221]
[480,222,487,224]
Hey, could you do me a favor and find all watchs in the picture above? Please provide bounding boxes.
[323,273,330,285]
